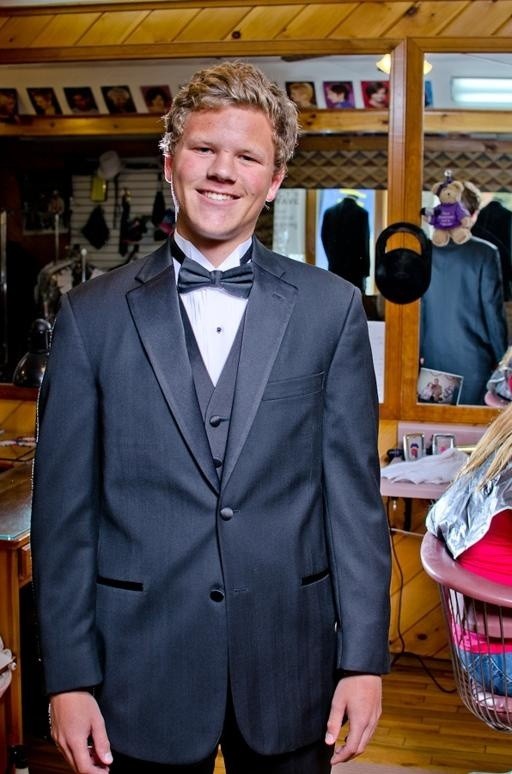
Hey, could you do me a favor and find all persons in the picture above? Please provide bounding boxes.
[28,57,396,773]
[450,376,463,405]
[103,86,133,114]
[415,178,509,408]
[324,80,356,111]
[320,193,371,298]
[444,400,512,699]
[429,378,443,402]
[288,82,315,109]
[361,78,388,107]
[143,86,173,113]
[0,90,18,112]
[30,90,61,117]
[67,88,95,115]
[442,377,457,406]
[418,381,432,401]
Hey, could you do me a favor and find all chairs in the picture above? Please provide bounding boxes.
[420,530,512,737]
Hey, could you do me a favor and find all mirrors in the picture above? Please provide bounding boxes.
[1,36,512,425]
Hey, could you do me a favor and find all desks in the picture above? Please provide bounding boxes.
[1,432,35,746]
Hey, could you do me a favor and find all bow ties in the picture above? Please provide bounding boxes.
[177,258,252,299]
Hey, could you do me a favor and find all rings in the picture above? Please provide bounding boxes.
[55,745,64,753]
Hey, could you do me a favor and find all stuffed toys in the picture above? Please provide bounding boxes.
[421,178,474,247]
